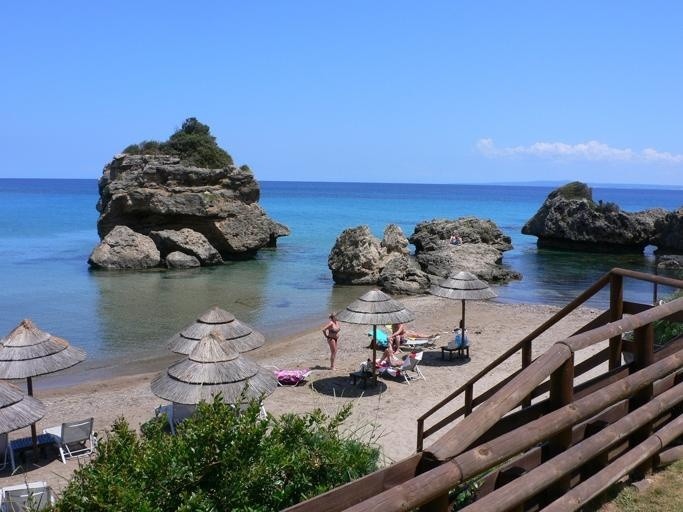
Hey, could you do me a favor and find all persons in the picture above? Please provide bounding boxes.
[388,323,407,350]
[378,343,415,366]
[321,312,340,371]
[448,231,462,246]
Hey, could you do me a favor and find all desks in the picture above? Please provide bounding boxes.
[440,346,469,360]
[349,371,378,389]
[10,433,55,464]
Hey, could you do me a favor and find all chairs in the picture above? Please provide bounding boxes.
[0,433,9,471]
[385,325,440,350]
[155,401,196,435]
[368,328,428,354]
[360,351,426,386]
[43,417,94,464]
[0,480,56,512]
